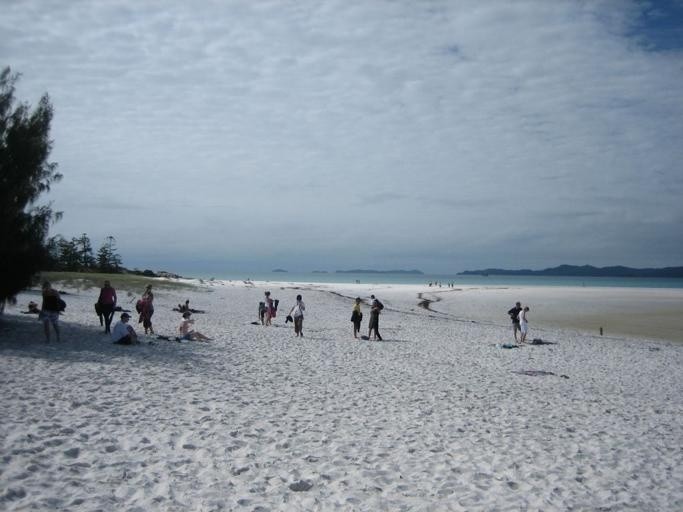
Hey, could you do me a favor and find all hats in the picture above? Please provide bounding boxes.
[121,313,132,319]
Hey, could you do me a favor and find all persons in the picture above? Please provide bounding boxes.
[506,302,521,342]
[38,281,62,344]
[141,284,154,334]
[263,290,276,327]
[177,311,214,343]
[111,313,139,346]
[96,278,117,333]
[366,294,381,339]
[258,301,268,325]
[288,294,305,337]
[351,297,361,338]
[367,301,384,341]
[20,305,40,314]
[515,306,529,342]
[177,300,204,314]
